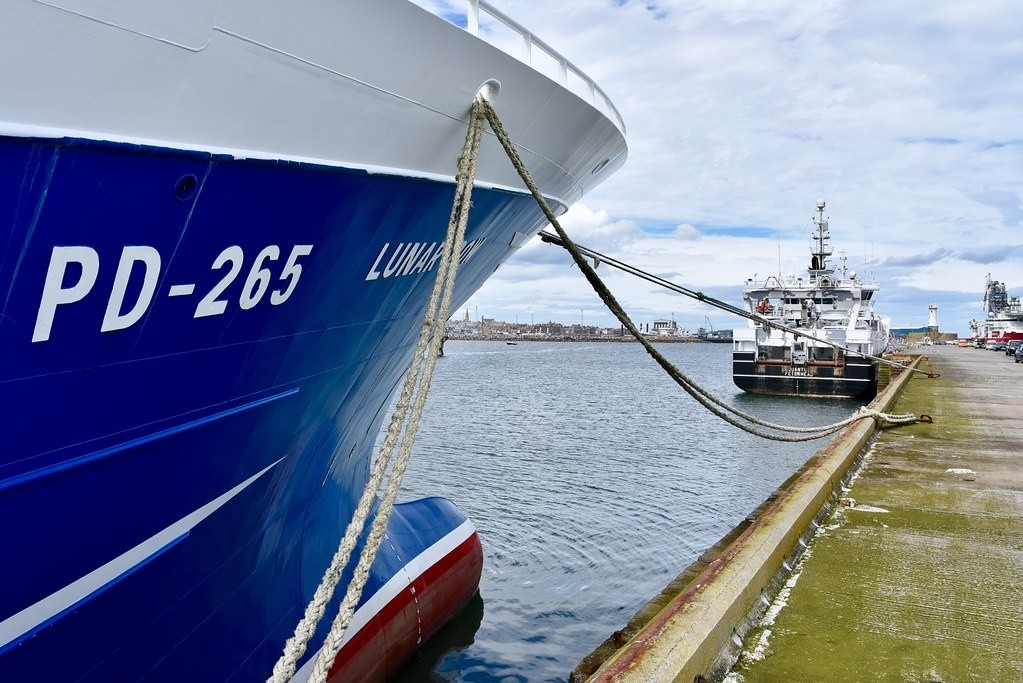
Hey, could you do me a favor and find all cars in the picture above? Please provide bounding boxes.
[985,340,996,350]
[994,342,1006,351]
[951,339,985,349]
[1013,344,1023,363]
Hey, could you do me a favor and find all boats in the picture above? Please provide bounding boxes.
[638,312,692,338]
[506,341,516,345]
[969,272,1023,344]
[731,196,890,402]
[0,0,628,683]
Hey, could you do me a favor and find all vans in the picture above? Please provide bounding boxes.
[923,337,931,346]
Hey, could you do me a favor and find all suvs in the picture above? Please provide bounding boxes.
[1005,340,1023,356]
